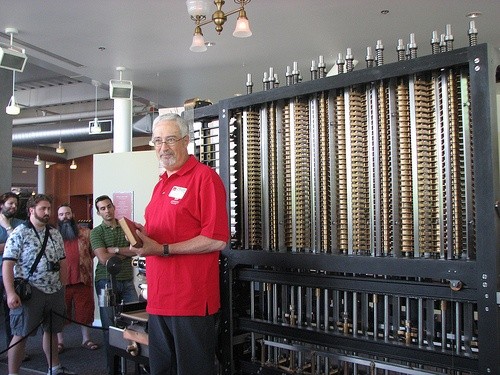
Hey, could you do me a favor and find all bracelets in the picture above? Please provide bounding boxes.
[163,244,169,257]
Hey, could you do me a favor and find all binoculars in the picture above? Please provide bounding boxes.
[47,261,60,271]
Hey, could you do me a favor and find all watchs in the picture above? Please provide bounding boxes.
[114,247,120,255]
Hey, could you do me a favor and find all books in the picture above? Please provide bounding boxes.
[118,216,143,248]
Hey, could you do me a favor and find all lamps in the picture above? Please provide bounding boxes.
[69,140,78,171]
[56,81,66,154]
[89,80,103,135]
[5,69,21,116]
[32,109,43,166]
[186,0,255,53]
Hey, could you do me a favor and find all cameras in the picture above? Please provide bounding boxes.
[47,261,60,271]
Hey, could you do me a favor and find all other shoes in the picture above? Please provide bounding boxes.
[0,351,8,362]
[22,354,30,362]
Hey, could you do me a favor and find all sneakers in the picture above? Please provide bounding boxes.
[46,363,80,375]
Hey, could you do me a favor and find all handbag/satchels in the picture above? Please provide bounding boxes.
[2,277,32,303]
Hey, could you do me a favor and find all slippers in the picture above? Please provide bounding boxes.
[58,342,64,354]
[82,340,98,350]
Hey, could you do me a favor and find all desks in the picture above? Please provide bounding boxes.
[107,311,149,375]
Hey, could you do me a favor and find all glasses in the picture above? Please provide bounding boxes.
[95,195,109,203]
[151,134,188,147]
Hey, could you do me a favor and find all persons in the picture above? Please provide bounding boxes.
[2,194,77,375]
[0,192,28,361]
[54,202,99,354]
[124,113,228,375]
[90,195,139,375]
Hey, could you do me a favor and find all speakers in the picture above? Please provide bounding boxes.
[109,82,132,99]
[0,46,28,72]
